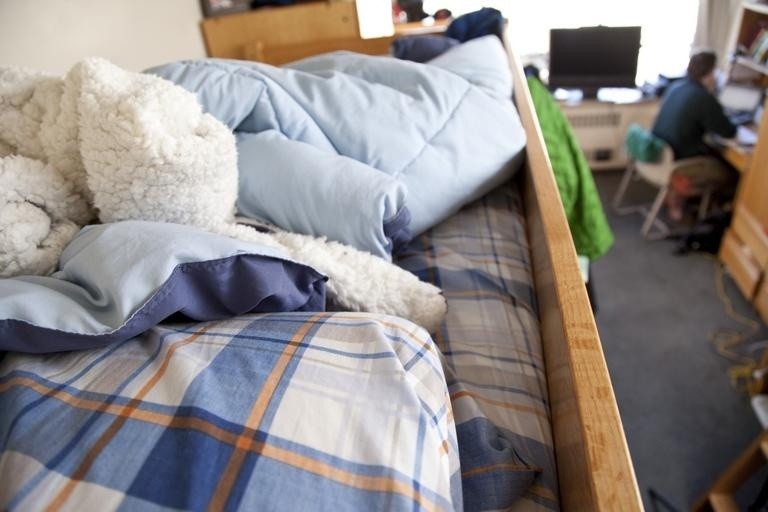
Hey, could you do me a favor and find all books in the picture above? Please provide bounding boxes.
[744,26,768,65]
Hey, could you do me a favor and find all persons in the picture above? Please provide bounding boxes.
[646,49,740,223]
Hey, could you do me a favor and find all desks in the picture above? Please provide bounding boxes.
[556,98,661,171]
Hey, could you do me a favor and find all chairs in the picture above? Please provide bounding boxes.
[614,124,728,242]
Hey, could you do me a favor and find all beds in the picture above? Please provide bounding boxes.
[1,8,645,512]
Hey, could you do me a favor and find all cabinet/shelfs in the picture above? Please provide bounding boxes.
[720,1,768,325]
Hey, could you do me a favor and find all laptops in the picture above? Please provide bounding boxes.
[717,83,763,125]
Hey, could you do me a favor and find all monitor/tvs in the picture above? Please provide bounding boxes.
[549,26,642,100]
